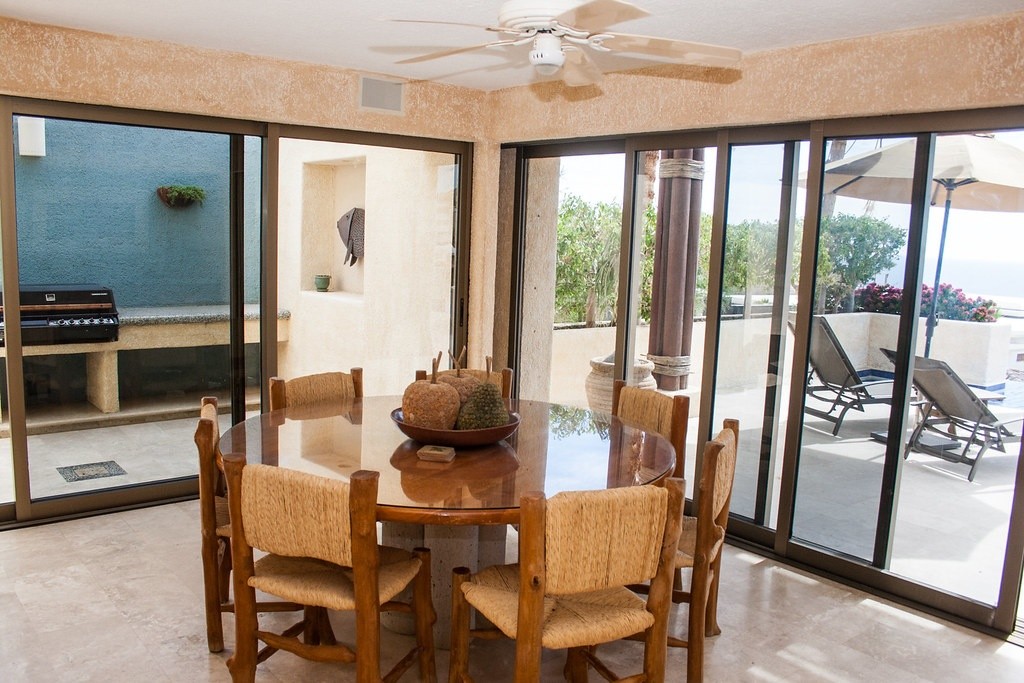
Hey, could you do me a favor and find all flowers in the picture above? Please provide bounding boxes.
[853,282,998,322]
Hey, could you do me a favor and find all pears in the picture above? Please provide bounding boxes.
[456,355,509,429]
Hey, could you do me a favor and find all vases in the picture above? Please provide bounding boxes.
[314,275,331,292]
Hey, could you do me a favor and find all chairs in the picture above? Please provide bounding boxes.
[612,380,690,478]
[447,478,686,683]
[221,453,438,683]
[787,312,1006,437]
[588,418,740,683]
[269,367,363,411]
[193,396,322,653]
[879,348,1024,482]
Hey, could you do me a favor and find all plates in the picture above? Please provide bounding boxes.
[390,405,524,449]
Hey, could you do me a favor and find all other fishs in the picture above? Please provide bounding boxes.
[337,208,364,266]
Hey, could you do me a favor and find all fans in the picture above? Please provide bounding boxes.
[391,0,743,88]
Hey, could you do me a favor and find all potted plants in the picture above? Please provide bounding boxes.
[586,219,657,415]
[157,186,207,209]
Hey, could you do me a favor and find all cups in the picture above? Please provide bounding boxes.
[314,275,332,292]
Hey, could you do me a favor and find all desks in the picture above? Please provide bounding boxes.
[215,395,676,650]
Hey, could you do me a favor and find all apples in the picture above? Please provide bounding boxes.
[402,345,482,429]
